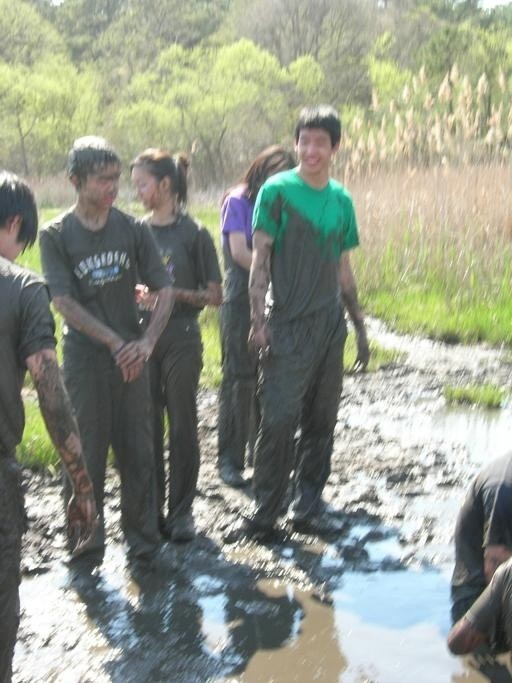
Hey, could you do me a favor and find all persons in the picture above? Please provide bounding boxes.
[0,166,105,683]
[446,446,512,656]
[442,554,512,658]
[217,99,373,545]
[116,145,223,543]
[35,133,182,590]
[217,144,304,488]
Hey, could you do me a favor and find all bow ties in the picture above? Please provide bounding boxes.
[127,454,349,559]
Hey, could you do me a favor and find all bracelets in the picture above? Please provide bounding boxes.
[111,339,130,358]
